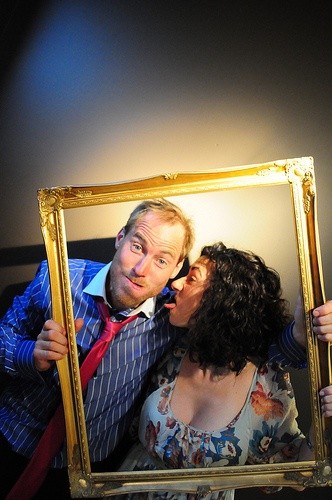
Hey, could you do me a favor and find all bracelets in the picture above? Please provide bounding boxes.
[305,433,313,453]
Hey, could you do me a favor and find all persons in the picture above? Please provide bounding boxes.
[0,201,331,500]
[109,239,332,500]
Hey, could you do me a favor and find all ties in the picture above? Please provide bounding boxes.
[3,295,137,500]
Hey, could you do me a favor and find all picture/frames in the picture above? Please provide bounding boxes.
[37,156,332,499]
